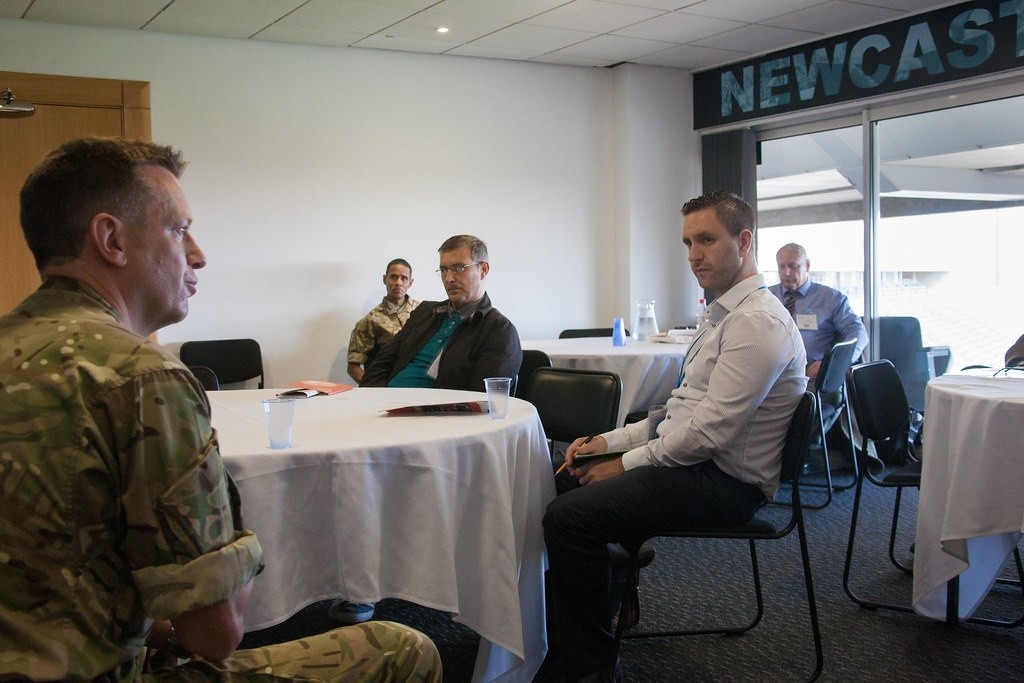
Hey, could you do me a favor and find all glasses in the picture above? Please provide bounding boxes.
[436,263,475,278]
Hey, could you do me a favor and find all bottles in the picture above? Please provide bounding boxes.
[696,299,708,329]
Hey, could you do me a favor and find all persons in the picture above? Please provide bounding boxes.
[0,137,445,683]
[767,243,869,479]
[347,259,422,384]
[330,234,523,622]
[542,191,805,682]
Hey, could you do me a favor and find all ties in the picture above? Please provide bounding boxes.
[784,294,796,315]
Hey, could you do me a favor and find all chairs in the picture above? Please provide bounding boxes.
[769,337,860,509]
[862,315,950,413]
[179,338,264,390]
[591,391,823,683]
[844,356,1024,627]
[528,368,624,492]
[511,349,553,401]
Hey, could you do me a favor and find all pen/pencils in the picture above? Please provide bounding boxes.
[554,432,595,478]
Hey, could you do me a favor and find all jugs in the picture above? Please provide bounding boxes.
[633,299,660,342]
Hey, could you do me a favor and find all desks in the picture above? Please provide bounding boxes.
[203,388,559,683]
[518,328,692,429]
[913,368,1024,624]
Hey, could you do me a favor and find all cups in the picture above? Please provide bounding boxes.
[483,377,513,418]
[262,398,296,450]
[613,317,626,346]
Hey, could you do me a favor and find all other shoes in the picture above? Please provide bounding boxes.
[325,598,374,624]
[803,459,811,476]
[571,656,623,683]
